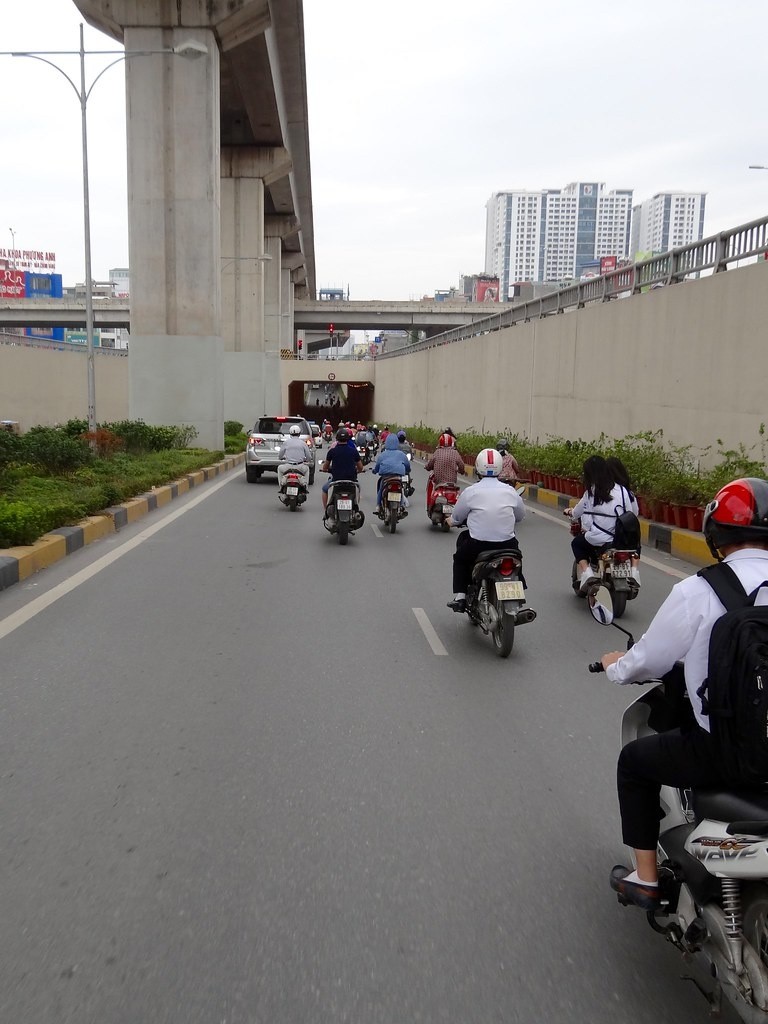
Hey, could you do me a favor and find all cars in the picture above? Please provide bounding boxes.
[310,424,324,449]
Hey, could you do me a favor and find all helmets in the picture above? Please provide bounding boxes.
[445,427,451,434]
[334,429,349,442]
[701,478,768,548]
[289,425,301,435]
[384,425,389,429]
[440,433,453,447]
[373,424,378,429]
[324,419,367,430]
[348,429,353,437]
[475,448,503,476]
[368,425,372,428]
[496,438,511,452]
[397,431,406,438]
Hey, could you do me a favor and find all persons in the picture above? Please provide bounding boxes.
[372,434,412,511]
[600,478,767,906]
[564,455,641,590]
[425,427,465,513]
[316,386,340,412]
[497,439,519,488]
[321,419,413,460]
[589,586,613,624]
[445,449,525,609]
[322,431,363,520]
[277,425,312,493]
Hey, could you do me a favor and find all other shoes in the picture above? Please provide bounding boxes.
[447,598,468,611]
[572,579,581,592]
[373,511,380,515]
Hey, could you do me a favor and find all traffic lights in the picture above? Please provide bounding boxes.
[329,324,334,337]
[298,339,303,350]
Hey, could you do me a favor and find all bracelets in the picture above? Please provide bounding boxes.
[568,510,572,515]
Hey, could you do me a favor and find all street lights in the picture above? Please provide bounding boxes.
[0,20,210,462]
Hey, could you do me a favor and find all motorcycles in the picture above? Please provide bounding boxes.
[321,431,414,467]
[317,458,366,547]
[582,577,768,1024]
[275,455,314,512]
[440,513,538,659]
[370,467,416,534]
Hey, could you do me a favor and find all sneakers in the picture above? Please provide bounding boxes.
[628,567,642,588]
[580,567,595,592]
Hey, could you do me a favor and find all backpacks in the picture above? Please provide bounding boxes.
[698,561,768,791]
[585,485,641,553]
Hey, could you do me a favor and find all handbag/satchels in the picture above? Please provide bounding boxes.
[402,488,410,509]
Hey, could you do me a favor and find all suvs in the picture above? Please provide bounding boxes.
[244,413,317,486]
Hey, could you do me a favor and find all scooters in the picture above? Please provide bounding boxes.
[475,469,518,491]
[561,507,641,620]
[422,463,465,532]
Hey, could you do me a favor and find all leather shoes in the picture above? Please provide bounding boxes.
[610,864,657,909]
